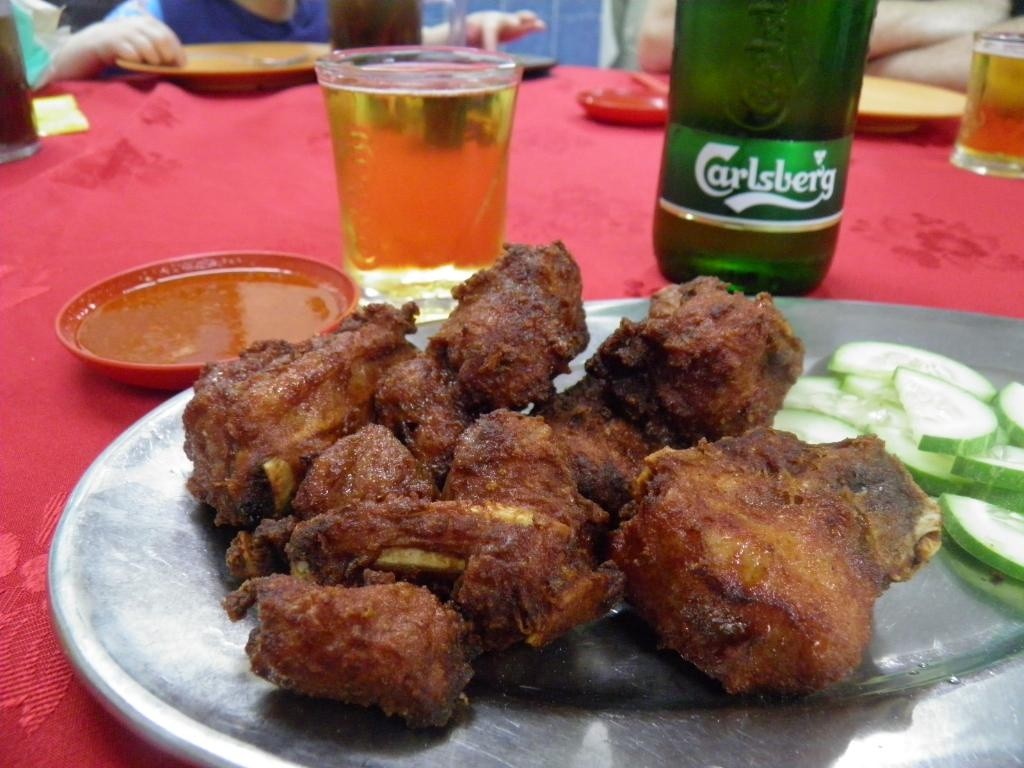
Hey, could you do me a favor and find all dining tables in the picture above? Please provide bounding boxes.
[3,61,1023,765]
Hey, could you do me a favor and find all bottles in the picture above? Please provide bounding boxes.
[651,1,876,301]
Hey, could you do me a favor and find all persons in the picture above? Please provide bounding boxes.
[48,1,544,85]
[637,0,1024,93]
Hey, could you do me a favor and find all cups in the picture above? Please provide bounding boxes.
[949,27,1024,179]
[316,38,527,326]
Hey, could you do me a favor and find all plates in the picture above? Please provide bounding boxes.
[46,295,1021,768]
[116,26,332,93]
[520,53,561,77]
[576,85,668,126]
[851,70,970,134]
[56,253,357,386]
